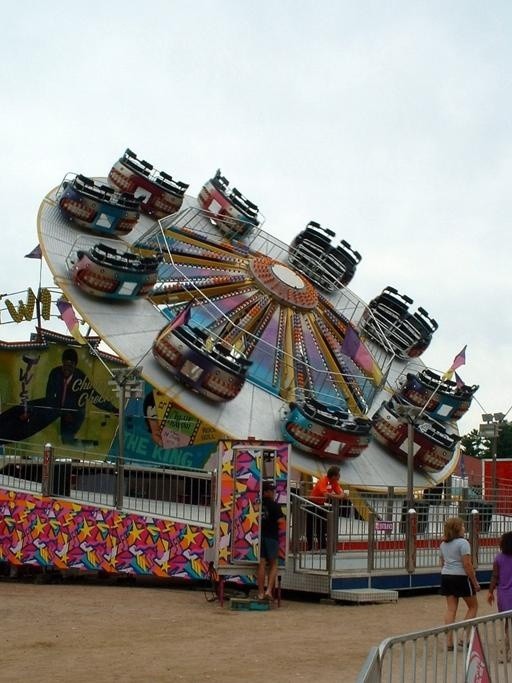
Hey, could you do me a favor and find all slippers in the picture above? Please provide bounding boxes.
[253,593,274,601]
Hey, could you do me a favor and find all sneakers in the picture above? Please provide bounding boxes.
[448,644,454,651]
[457,639,469,648]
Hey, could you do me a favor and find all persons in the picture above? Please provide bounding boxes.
[438,516,482,651]
[487,531,512,665]
[44,347,118,447]
[143,391,163,447]
[254,483,286,601]
[305,466,347,551]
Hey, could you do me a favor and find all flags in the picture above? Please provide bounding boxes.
[56,297,87,345]
[338,325,394,394]
[156,305,195,344]
[442,348,467,380]
[455,373,465,390]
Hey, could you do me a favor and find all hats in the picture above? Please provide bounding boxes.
[263,481,277,490]
